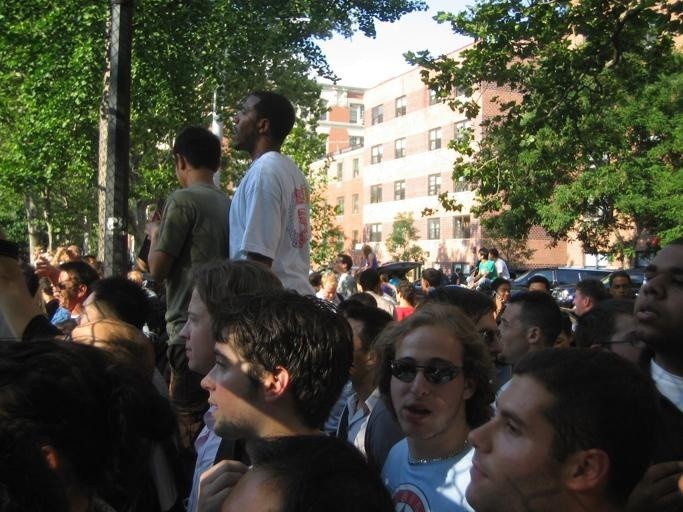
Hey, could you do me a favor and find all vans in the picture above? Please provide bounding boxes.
[503,267,611,294]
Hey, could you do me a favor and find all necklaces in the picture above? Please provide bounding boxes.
[408,436,470,467]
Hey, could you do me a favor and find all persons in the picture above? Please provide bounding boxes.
[339,304,403,473]
[526,275,552,294]
[371,298,500,512]
[466,247,498,292]
[487,248,511,282]
[27,244,100,337]
[197,433,396,512]
[363,245,380,272]
[230,90,317,298]
[308,267,342,310]
[420,267,443,299]
[1,338,146,512]
[575,297,654,371]
[74,274,154,331]
[388,270,405,290]
[449,267,470,287]
[200,289,354,471]
[143,126,232,453]
[423,286,503,370]
[489,276,512,323]
[336,292,378,317]
[308,271,323,294]
[177,258,287,511]
[1,226,157,385]
[394,279,418,322]
[497,289,563,379]
[622,235,683,512]
[465,346,664,512]
[360,267,398,319]
[608,270,635,298]
[332,253,358,302]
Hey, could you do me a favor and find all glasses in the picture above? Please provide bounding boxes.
[390,357,472,383]
[57,282,67,290]
[605,335,647,350]
[482,330,503,344]
